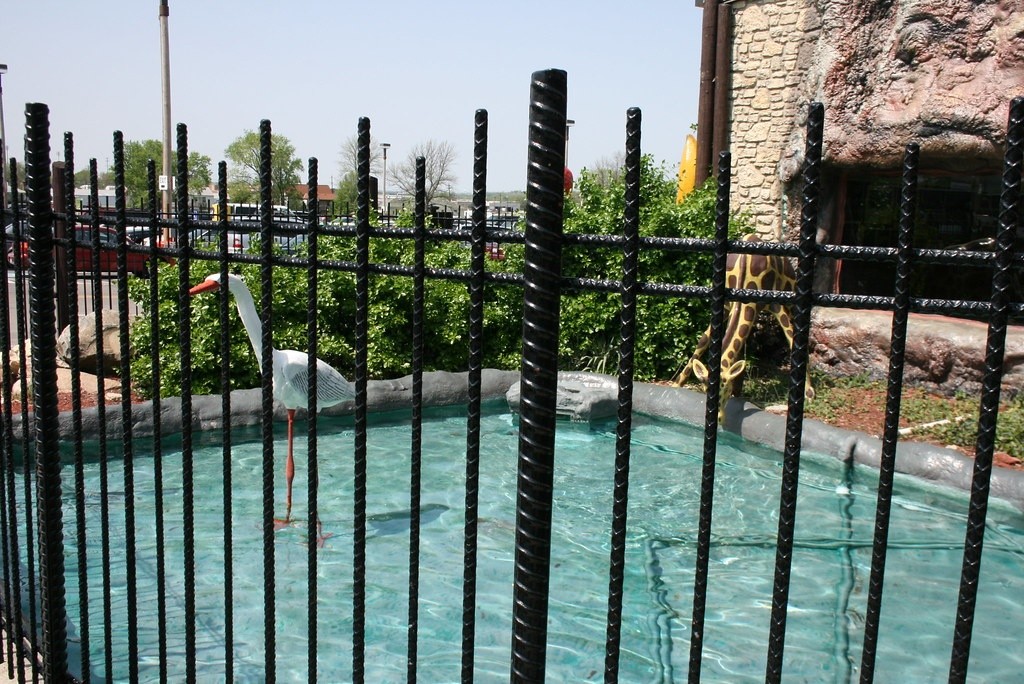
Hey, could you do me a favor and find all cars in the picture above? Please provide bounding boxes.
[0,188,319,257]
[293,211,324,226]
[7,224,175,280]
[332,217,357,227]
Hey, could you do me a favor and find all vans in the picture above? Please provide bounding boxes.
[222,203,304,225]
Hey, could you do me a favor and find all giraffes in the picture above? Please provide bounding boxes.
[670,232,816,425]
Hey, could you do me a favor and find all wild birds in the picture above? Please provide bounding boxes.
[185,271,357,549]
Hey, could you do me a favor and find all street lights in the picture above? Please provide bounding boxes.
[0,63,10,209]
[380,143,391,226]
